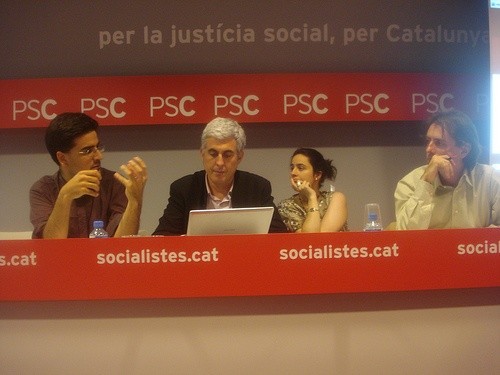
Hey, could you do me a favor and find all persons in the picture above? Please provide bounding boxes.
[151,118,287,239]
[277,148,349,233]
[29,112,149,240]
[394,110,500,231]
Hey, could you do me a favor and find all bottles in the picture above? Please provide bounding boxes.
[364,214,383,231]
[89,221,109,238]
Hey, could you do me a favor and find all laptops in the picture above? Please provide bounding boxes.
[186,207,274,237]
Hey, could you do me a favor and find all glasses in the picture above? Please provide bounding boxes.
[63,143,106,156]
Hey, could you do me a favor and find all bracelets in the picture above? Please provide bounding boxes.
[306,208,320,212]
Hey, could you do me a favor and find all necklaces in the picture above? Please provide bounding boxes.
[296,193,324,212]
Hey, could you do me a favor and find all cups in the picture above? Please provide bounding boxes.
[362,204,384,232]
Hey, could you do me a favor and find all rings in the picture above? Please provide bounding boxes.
[297,182,301,187]
